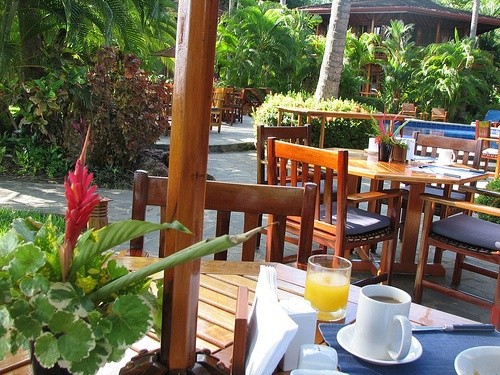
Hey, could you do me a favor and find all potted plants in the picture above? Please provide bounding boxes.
[368,110,413,157]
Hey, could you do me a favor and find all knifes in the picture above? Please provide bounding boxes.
[410,323,496,333]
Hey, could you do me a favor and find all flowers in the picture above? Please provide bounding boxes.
[0,124,279,375]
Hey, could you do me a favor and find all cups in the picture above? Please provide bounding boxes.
[438,149,456,162]
[355,283,412,361]
[369,138,378,151]
[304,254,353,321]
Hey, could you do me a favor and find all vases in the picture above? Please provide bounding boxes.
[392,145,407,161]
[29,326,74,375]
[378,143,391,161]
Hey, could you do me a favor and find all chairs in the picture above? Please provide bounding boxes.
[210,86,245,132]
[400,103,417,119]
[471,110,500,127]
[431,108,447,122]
[128,123,500,331]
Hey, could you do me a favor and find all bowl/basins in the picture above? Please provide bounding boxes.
[452,345,500,375]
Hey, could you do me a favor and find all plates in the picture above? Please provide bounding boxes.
[364,149,379,155]
[336,323,423,366]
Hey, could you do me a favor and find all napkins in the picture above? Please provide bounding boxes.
[318,323,500,375]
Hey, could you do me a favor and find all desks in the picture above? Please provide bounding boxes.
[298,145,490,276]
[0,257,500,375]
[276,106,414,149]
